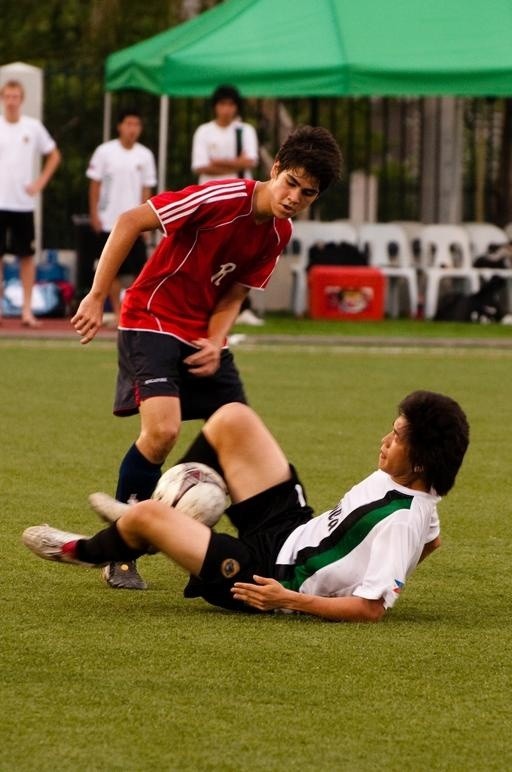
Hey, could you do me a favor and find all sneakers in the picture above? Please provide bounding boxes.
[101,559,147,590]
[23,525,110,569]
[87,492,132,524]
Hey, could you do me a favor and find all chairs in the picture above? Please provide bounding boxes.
[290,220,512,320]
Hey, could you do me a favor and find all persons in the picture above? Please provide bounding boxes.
[190,83,269,332]
[70,122,344,593]
[0,79,62,330]
[21,387,470,620]
[85,104,158,317]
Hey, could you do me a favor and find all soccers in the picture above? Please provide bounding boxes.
[155,463,228,526]
